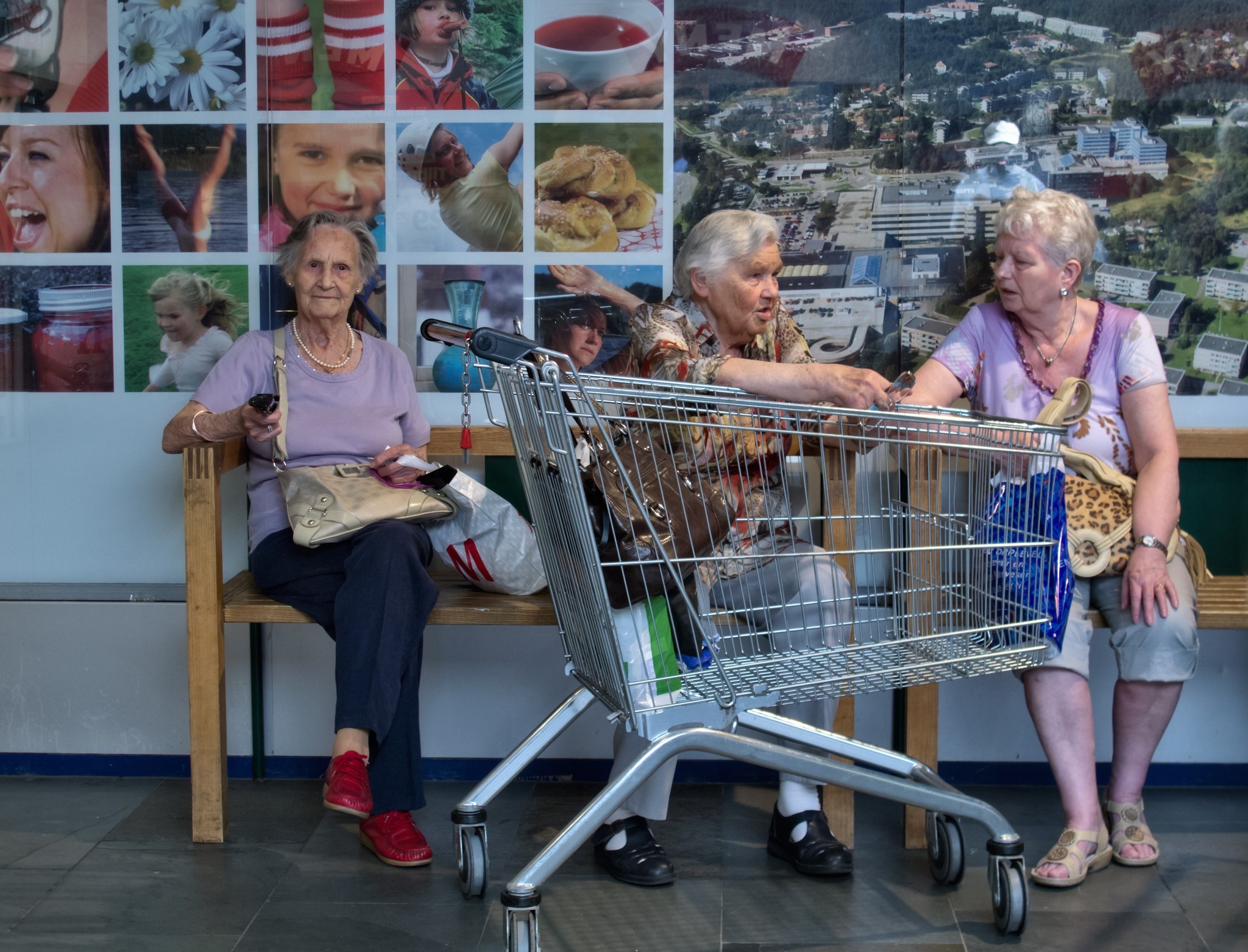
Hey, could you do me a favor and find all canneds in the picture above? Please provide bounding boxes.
[0,284,114,393]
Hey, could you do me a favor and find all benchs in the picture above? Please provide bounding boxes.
[183,426,856,849]
[887,427,1248,853]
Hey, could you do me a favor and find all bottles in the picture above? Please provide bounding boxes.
[431,279,497,393]
[31,283,114,393]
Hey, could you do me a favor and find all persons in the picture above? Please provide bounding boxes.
[593,211,914,895]
[161,209,438,866]
[0,1,646,393]
[894,187,1203,888]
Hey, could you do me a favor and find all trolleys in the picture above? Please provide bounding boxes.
[415,314,1071,952]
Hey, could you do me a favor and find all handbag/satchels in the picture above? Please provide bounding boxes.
[533,347,736,610]
[972,433,1075,659]
[272,326,457,547]
[610,595,682,712]
[398,453,548,595]
[1004,376,1213,589]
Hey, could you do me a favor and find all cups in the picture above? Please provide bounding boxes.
[0,308,28,392]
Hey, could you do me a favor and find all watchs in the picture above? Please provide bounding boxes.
[1132,536,1168,556]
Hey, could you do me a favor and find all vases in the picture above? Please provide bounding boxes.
[431,280,498,393]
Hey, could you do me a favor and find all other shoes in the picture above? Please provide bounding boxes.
[765,801,853,874]
[321,750,374,818]
[590,816,677,885]
[355,811,434,867]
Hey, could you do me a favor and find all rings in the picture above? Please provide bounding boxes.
[267,425,272,432]
[1156,582,1165,590]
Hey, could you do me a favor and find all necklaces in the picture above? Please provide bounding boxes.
[292,316,355,367]
[316,325,349,374]
[1030,294,1078,368]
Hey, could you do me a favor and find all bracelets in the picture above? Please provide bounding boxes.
[192,411,218,440]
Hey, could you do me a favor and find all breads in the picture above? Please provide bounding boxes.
[534,145,656,252]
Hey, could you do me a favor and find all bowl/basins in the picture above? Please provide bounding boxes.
[533,0,664,100]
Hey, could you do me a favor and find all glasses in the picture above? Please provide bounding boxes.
[859,371,916,432]
[249,393,280,414]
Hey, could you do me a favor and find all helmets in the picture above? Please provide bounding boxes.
[397,121,443,181]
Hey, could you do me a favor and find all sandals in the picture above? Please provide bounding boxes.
[1032,826,1113,886]
[1105,797,1159,866]
